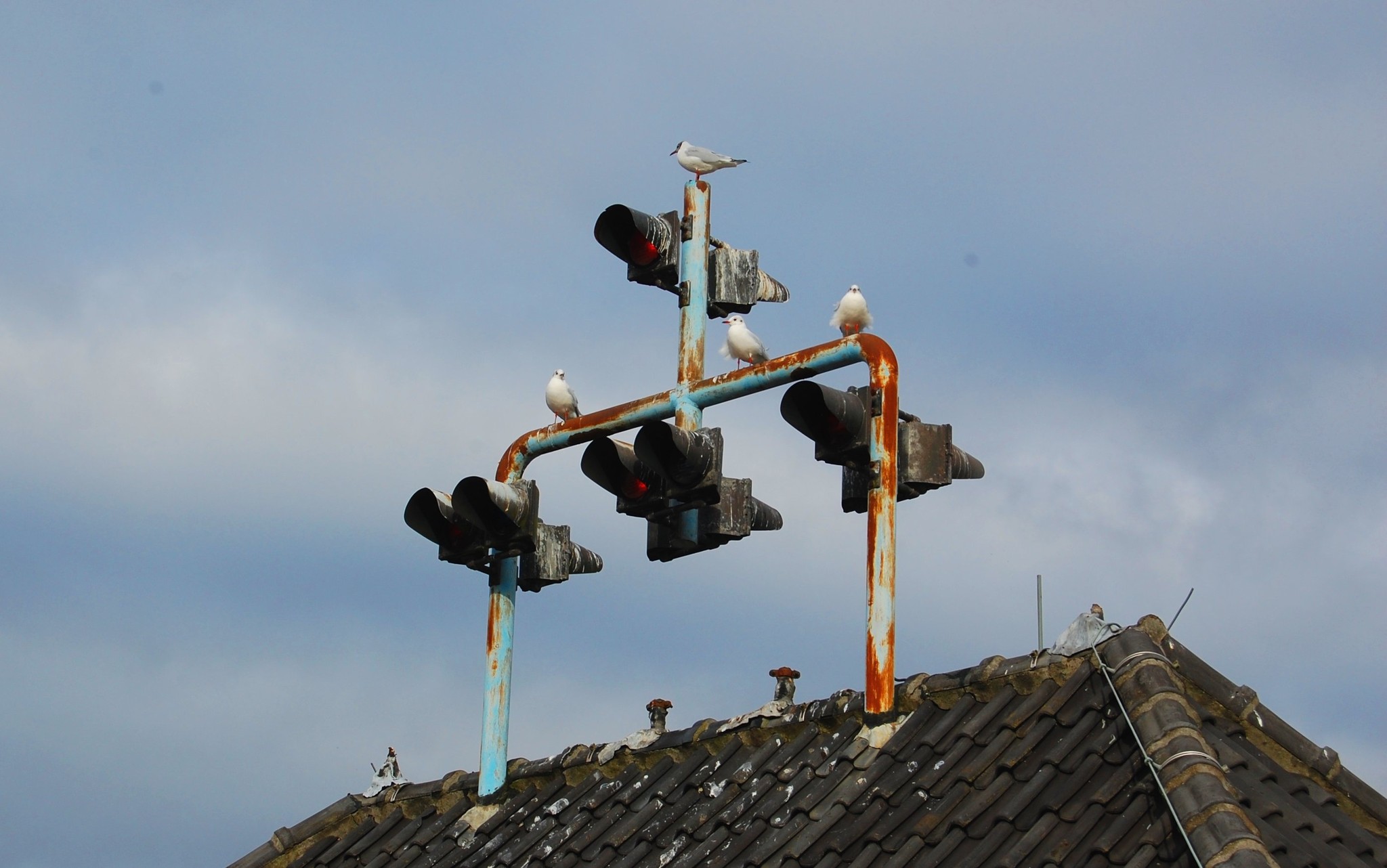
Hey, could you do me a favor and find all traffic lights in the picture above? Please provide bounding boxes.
[778,379,872,462]
[897,420,986,490]
[519,523,603,589]
[579,419,722,514]
[403,475,537,563]
[706,247,790,319]
[700,474,783,543]
[594,202,679,286]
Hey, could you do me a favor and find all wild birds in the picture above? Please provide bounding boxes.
[721,316,770,372]
[829,284,873,336]
[546,370,583,425]
